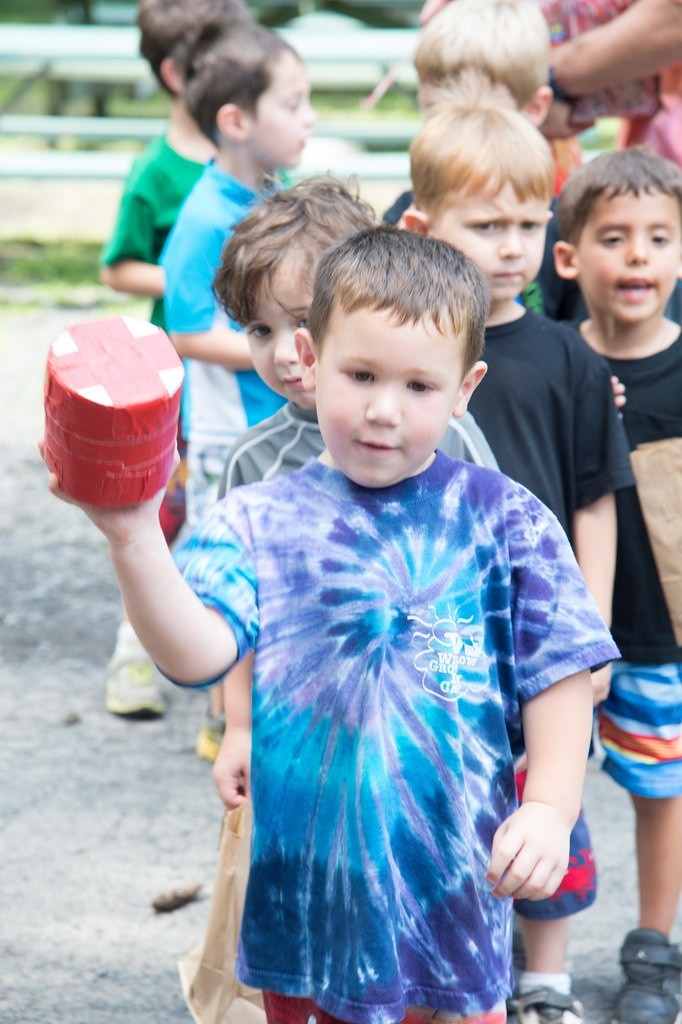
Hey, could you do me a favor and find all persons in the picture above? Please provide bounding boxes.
[384,1,682,331]
[178,180,501,1024]
[161,25,316,760]
[409,92,633,1024]
[553,148,682,1024]
[38,228,621,1024]
[99,0,246,715]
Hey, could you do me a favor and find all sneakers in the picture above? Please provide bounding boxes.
[613,925,682,1024]
[505,978,583,1024]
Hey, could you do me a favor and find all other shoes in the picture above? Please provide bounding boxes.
[104,621,165,718]
[195,708,227,761]
